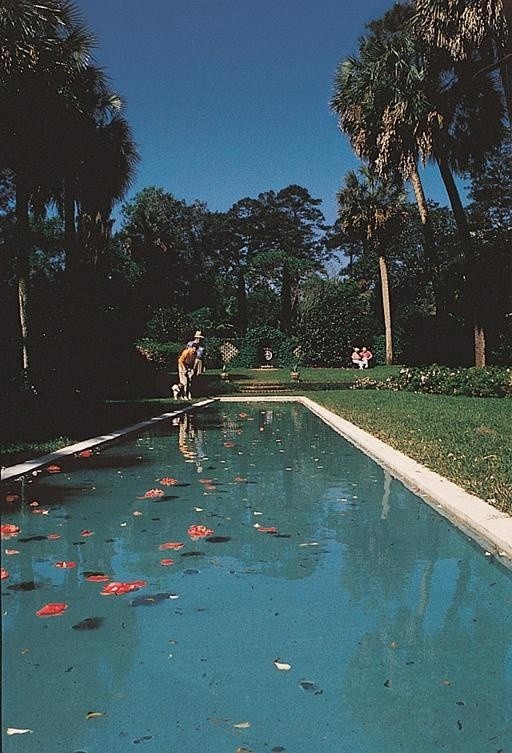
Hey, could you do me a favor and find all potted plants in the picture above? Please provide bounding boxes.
[220,363,231,380]
[289,356,301,380]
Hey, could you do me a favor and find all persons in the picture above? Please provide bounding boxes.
[170,342,197,400]
[177,413,200,465]
[351,347,365,368]
[187,330,207,376]
[191,412,206,474]
[359,346,372,368]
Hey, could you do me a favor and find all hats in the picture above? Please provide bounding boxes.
[194,331,204,339]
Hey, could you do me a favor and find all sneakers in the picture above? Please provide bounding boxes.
[183,395,192,401]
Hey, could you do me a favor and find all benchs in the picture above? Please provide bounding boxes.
[343,353,377,368]
